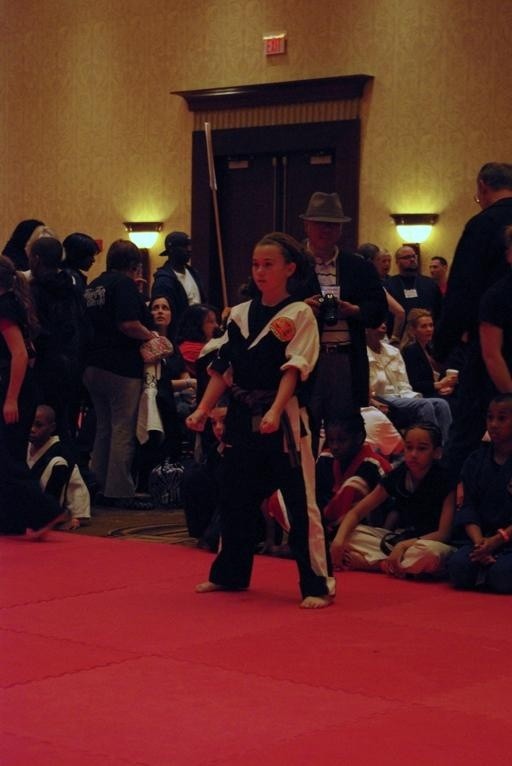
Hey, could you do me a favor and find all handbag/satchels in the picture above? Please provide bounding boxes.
[140,330,174,365]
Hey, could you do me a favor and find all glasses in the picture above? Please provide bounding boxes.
[473,194,480,204]
[399,254,417,260]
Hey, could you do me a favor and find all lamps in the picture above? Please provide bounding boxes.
[390,212,440,245]
[123,220,164,251]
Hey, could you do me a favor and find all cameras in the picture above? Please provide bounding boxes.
[316,293,340,326]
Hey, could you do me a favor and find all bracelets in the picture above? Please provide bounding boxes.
[497,528,510,542]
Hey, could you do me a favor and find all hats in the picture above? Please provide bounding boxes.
[158,232,192,256]
[298,192,353,224]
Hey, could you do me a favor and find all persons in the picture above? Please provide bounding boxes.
[186,233,335,608]
[136,294,197,446]
[26,404,91,530]
[131,259,147,294]
[388,246,447,337]
[29,237,87,438]
[317,409,398,549]
[381,252,391,281]
[63,233,101,290]
[0,255,37,535]
[429,256,449,295]
[357,243,405,348]
[445,162,512,464]
[365,322,453,447]
[179,392,290,555]
[247,191,388,475]
[327,421,456,579]
[1,220,54,271]
[399,308,464,419]
[81,239,159,509]
[173,304,218,368]
[318,388,404,457]
[151,232,209,312]
[450,395,512,594]
